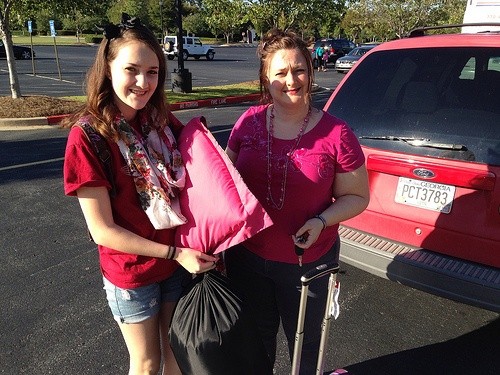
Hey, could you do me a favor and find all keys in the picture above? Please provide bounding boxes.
[295,238,306,267]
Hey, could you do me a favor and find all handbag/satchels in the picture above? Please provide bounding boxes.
[168,253,275,374]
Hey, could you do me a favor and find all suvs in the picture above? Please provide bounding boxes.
[319,24,500,313]
[163,35,215,61]
[314,39,358,65]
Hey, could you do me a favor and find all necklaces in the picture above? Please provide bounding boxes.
[266,104,312,210]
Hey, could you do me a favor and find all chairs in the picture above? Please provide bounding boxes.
[360,51,366,55]
[396,69,500,113]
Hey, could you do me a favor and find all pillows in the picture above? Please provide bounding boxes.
[171,115,275,257]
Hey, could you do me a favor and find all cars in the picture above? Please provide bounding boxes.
[334,46,376,74]
[0,39,36,60]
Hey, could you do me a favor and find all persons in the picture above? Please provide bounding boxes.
[224,27,370,375]
[60,12,219,375]
[316,44,329,72]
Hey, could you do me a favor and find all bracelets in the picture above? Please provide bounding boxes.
[311,215,327,232]
[166,244,176,261]
